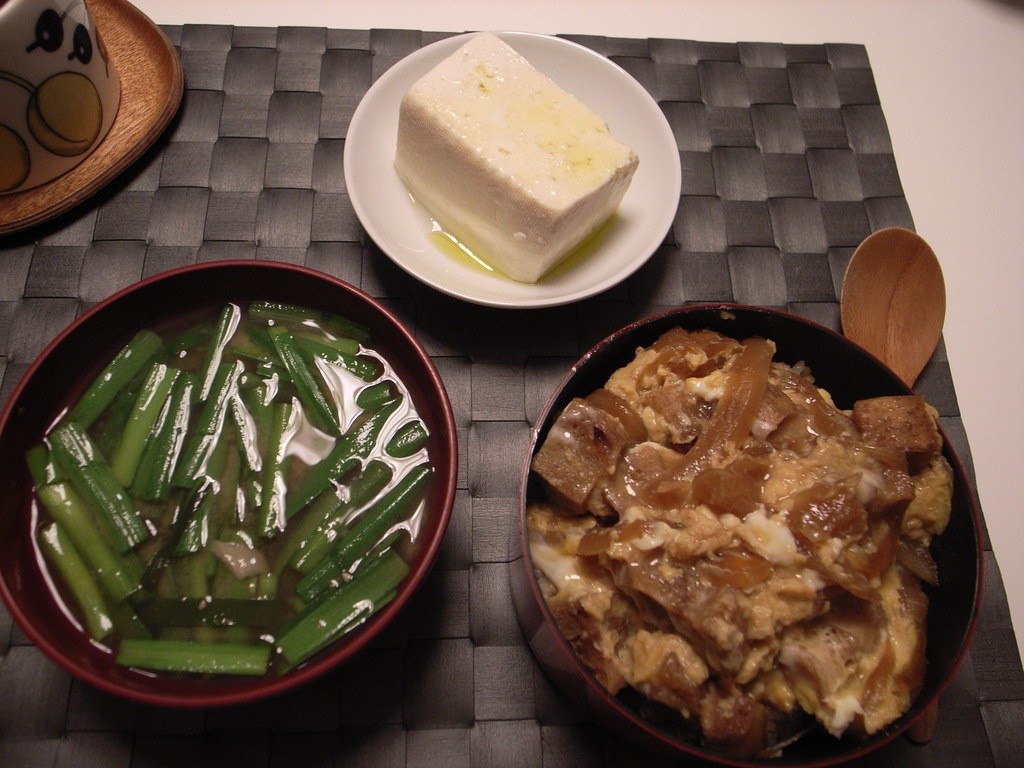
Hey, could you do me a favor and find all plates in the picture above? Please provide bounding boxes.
[343,32,683,310]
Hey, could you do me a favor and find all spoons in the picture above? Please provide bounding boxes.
[840,227,946,743]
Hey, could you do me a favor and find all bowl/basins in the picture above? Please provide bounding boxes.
[507,300,985,768]
[0,257,458,711]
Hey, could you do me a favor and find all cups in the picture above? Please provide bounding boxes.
[0,0,121,196]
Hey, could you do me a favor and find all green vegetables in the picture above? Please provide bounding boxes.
[27,301,434,677]
